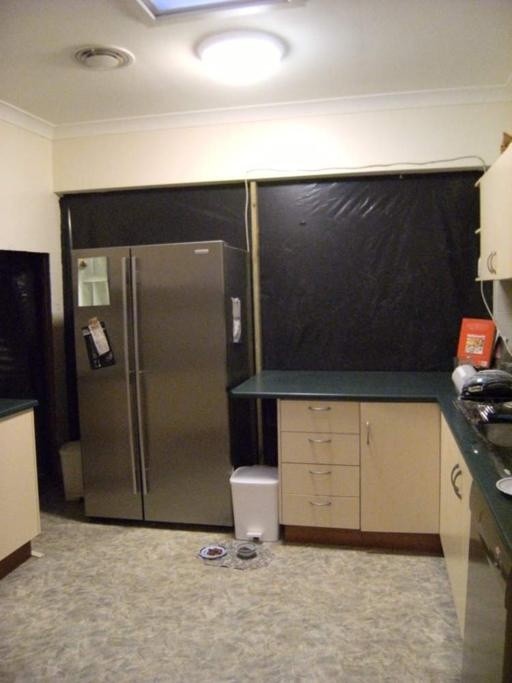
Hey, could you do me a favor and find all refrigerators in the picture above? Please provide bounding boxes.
[71,239,253,528]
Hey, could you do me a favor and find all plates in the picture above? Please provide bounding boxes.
[495,475,512,496]
[199,546,226,560]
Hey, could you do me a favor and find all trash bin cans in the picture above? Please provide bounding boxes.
[229,465,279,543]
[60,442,84,502]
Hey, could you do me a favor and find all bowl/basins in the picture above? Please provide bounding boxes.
[236,543,256,560]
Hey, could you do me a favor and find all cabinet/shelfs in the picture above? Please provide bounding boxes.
[0,407,42,581]
[439,409,474,641]
[276,398,441,554]
[473,144,512,281]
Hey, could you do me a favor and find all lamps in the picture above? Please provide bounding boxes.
[196,30,287,89]
